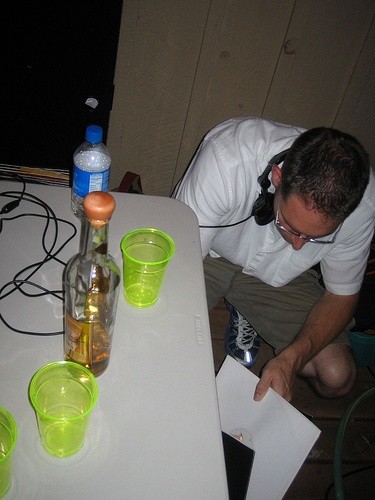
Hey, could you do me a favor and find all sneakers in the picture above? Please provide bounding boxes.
[223,297,260,368]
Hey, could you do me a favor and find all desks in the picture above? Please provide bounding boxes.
[0,180,231,500]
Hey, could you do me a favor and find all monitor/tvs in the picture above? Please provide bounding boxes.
[0,0,124,170]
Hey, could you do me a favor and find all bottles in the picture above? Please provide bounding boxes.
[61,192,115,379]
[70,125,113,219]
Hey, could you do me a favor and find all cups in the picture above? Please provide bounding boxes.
[29,360,98,457]
[120,228,174,308]
[0,406,18,498]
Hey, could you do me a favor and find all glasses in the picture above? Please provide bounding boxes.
[274,184,344,244]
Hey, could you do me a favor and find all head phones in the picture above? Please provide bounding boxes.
[251,147,290,226]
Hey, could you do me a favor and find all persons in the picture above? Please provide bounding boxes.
[168,117,375,402]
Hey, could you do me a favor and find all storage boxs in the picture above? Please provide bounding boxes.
[350,331,374,366]
